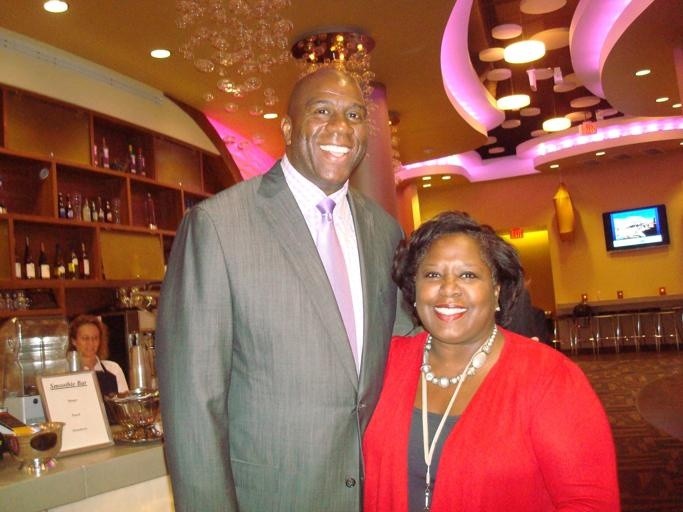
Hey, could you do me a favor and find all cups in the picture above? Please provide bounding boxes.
[67,191,82,220]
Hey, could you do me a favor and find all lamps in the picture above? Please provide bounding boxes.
[176,0,295,117]
[389,111,406,174]
[358,81,389,140]
[478,0,619,158]
[288,24,381,142]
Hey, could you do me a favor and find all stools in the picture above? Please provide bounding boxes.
[544,308,682,357]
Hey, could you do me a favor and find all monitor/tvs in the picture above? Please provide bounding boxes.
[602,205,671,251]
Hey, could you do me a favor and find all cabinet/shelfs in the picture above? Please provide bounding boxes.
[0,80,233,378]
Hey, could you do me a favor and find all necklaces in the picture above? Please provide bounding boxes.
[420,322,496,387]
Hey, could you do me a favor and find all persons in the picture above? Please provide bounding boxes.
[360,209,621,512]
[64,314,130,427]
[153,66,423,512]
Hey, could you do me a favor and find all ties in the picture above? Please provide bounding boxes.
[315,196,359,379]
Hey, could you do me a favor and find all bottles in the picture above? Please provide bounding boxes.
[15,245,22,280]
[52,242,90,279]
[66,200,74,219]
[24,235,36,280]
[97,193,105,223]
[100,136,110,168]
[128,145,136,174]
[58,191,66,219]
[37,242,50,280]
[91,201,97,220]
[137,147,146,177]
[184,199,192,213]
[82,198,92,221]
[104,200,112,223]
